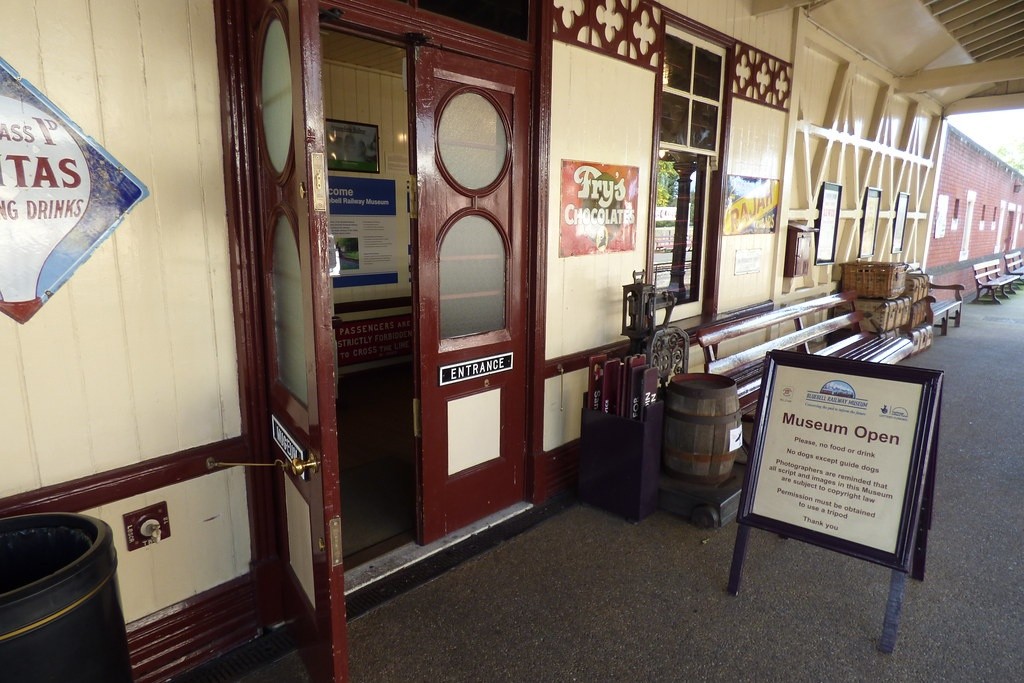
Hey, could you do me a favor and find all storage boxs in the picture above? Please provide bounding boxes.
[833,274,929,334]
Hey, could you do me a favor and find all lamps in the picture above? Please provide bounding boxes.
[1014,178,1022,193]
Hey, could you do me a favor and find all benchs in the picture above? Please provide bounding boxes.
[971,251,1024,305]
[907,269,965,336]
[697,289,914,466]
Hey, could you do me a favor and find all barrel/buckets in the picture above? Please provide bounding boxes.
[660,372,741,485]
